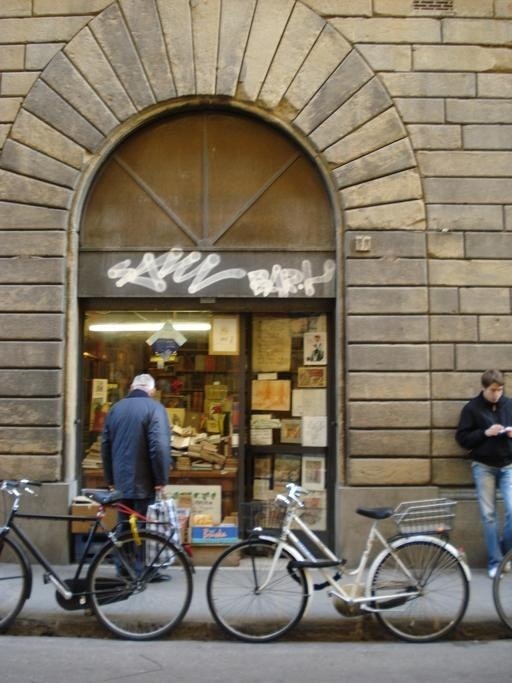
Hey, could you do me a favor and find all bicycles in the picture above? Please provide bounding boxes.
[491,547,512,632]
[206,481,473,644]
[0,479,192,640]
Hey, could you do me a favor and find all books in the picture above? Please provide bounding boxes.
[81,436,102,469]
[171,353,240,475]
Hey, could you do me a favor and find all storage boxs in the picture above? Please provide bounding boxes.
[71,502,118,534]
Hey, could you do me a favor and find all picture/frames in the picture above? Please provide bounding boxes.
[303,332,327,365]
[208,314,240,356]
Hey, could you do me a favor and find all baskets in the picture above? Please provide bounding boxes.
[391,497,460,536]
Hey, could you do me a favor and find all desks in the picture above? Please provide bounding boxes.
[83,458,239,516]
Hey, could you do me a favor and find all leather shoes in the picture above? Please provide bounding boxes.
[149,573,172,583]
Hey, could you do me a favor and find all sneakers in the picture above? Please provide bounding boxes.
[488,568,503,579]
[502,560,511,572]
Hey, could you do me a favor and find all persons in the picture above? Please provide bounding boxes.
[455,369,512,578]
[101,374,171,583]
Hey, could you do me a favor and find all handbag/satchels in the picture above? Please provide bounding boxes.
[142,498,184,569]
